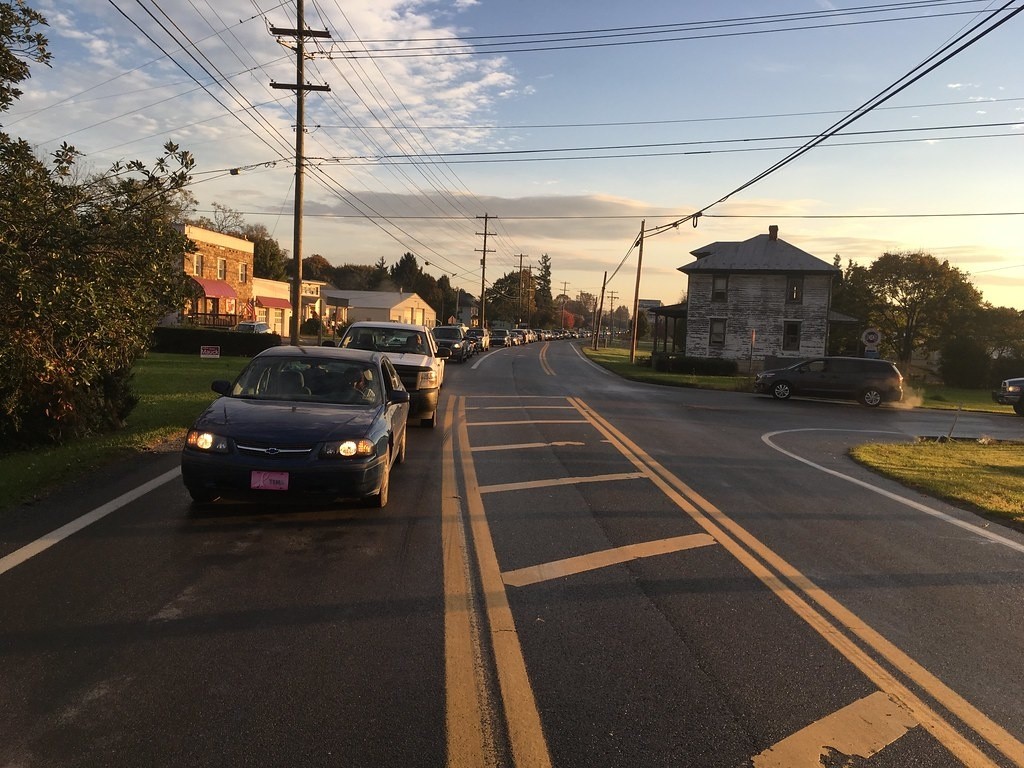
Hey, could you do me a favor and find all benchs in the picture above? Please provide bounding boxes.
[375,335,425,352]
[303,368,346,393]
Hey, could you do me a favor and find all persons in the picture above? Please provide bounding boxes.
[334,368,375,402]
[402,334,426,354]
[467,331,479,355]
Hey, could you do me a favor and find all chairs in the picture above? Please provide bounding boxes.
[355,333,378,349]
[821,361,828,372]
[403,336,419,352]
[267,371,311,395]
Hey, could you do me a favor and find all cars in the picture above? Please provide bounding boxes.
[233,320,272,334]
[179,342,413,517]
[336,318,454,431]
[753,350,905,407]
[991,374,1024,415]
[430,321,618,363]
